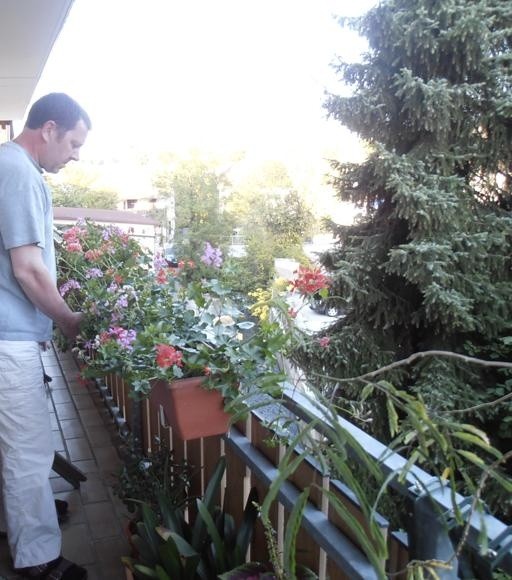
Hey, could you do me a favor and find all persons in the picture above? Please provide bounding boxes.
[0,93,95,577]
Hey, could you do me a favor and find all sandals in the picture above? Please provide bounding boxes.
[20,556,86,579]
